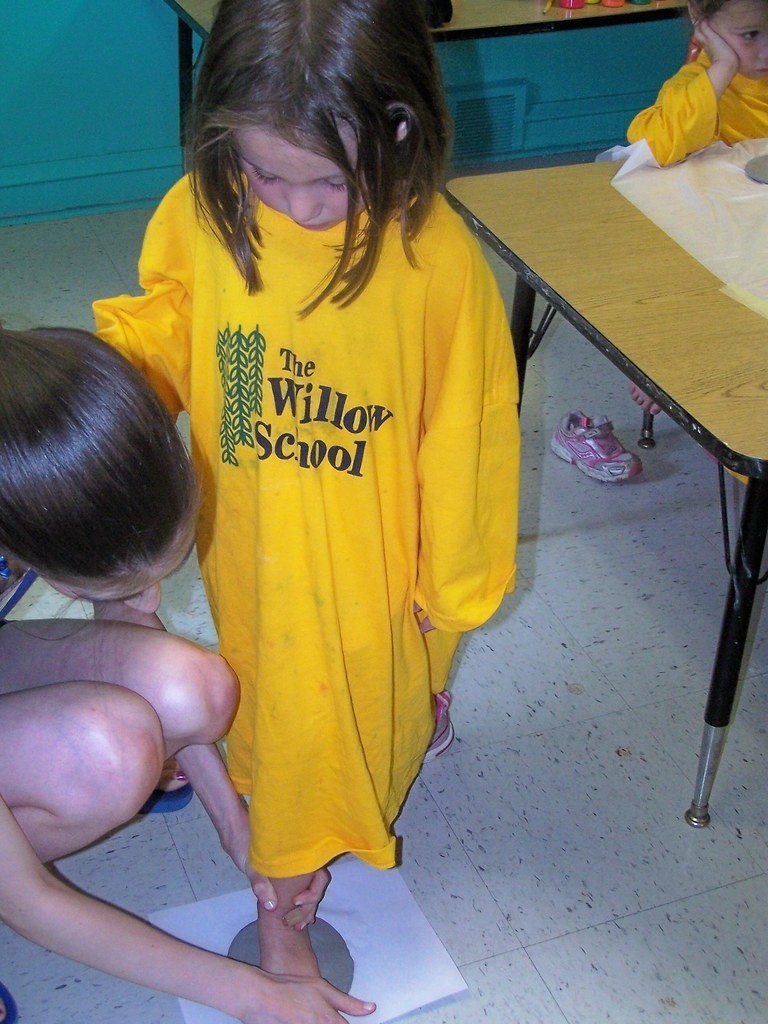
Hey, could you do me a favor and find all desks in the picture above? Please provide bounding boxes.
[168,2,719,159]
[438,149,768,828]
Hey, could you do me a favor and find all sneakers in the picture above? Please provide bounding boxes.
[550,408,642,482]
[422,691,456,766]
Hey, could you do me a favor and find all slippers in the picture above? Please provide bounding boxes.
[0,983,18,1024]
[138,782,193,814]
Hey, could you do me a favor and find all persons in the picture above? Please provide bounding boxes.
[91,1,522,981]
[625,0,768,415]
[0,322,379,1024]
